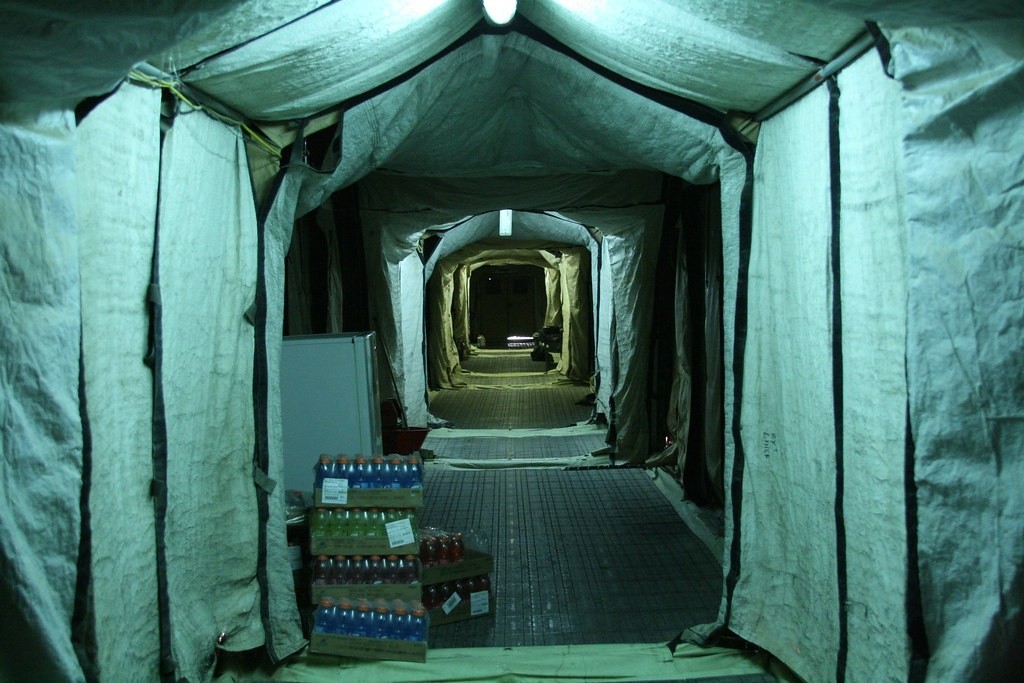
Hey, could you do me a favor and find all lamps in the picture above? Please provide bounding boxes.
[499,209,512,236]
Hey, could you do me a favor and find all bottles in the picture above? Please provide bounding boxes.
[314,598,426,641]
[315,555,420,584]
[311,507,417,537]
[428,575,491,609]
[289,492,305,507]
[421,529,463,566]
[315,454,423,488]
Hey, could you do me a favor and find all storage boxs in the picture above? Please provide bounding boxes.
[307,486,493,662]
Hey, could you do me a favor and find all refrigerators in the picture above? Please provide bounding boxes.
[280,331,383,489]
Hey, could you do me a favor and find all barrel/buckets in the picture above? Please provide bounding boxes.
[477,334,486,347]
